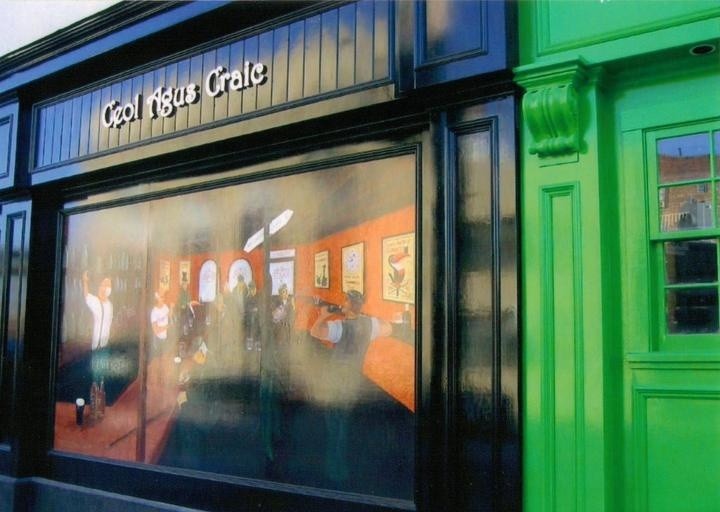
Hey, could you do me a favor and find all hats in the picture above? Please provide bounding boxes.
[181,272,188,281]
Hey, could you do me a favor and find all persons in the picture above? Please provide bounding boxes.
[83,269,113,382]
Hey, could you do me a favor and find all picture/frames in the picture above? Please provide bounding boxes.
[381,231,418,303]
[313,250,332,288]
[340,241,367,297]
